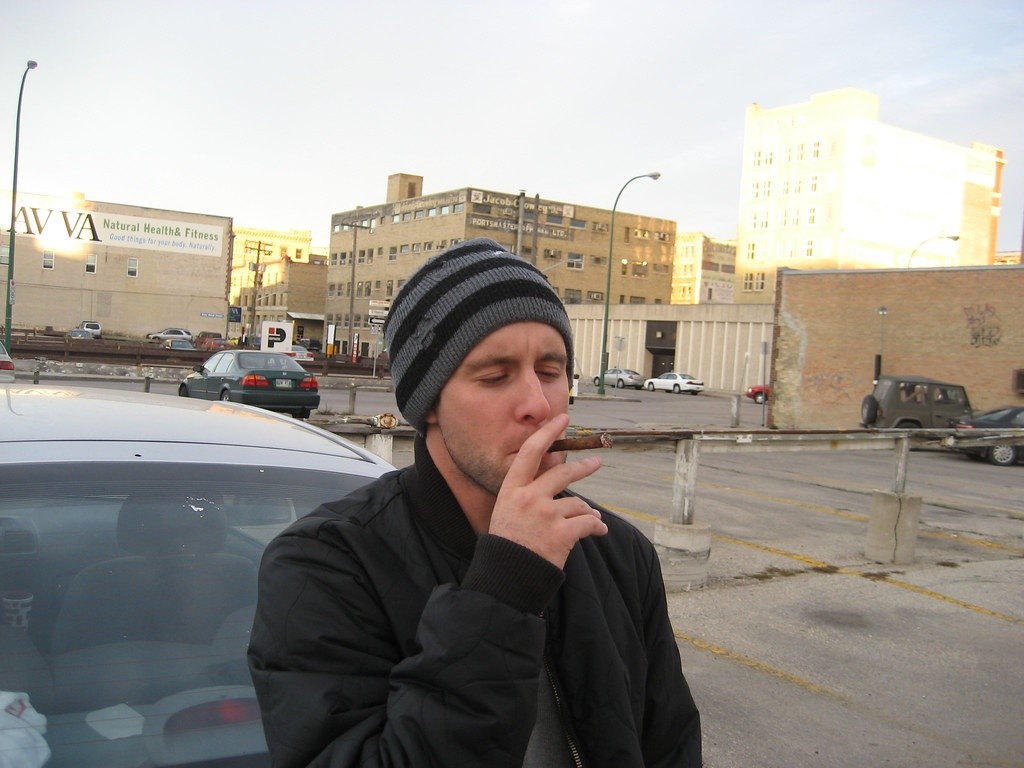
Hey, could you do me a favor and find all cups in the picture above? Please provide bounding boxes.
[1,591,33,634]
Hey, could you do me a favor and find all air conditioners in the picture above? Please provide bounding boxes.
[590,293,601,299]
[549,250,558,256]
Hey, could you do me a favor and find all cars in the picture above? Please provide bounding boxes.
[159,338,196,351]
[287,337,321,362]
[0,338,16,384]
[592,367,645,390]
[64,328,95,342]
[0,382,403,768]
[746,384,769,404]
[178,349,321,421]
[938,405,1024,467]
[860,372,975,451]
[643,371,704,395]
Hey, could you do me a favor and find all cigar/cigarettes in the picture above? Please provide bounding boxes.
[547,433,613,450]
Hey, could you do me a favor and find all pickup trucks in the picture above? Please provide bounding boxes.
[192,331,235,350]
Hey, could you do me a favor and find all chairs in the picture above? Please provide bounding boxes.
[54,492,262,654]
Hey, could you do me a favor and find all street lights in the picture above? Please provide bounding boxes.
[5,59,38,358]
[598,172,663,395]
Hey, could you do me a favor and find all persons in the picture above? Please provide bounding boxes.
[246,238,702,768]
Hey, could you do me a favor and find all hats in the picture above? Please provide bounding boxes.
[382,237,574,439]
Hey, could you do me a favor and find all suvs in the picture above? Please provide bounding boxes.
[76,320,102,339]
[147,328,193,345]
